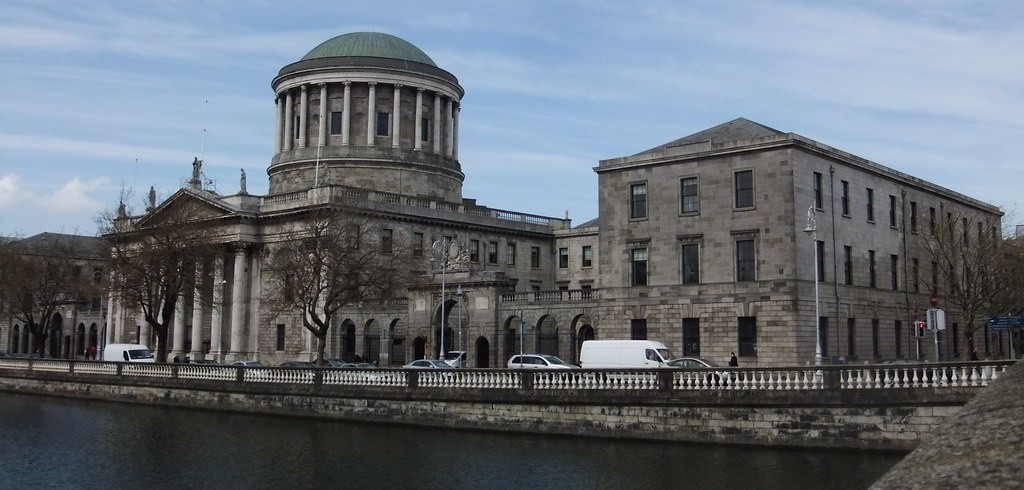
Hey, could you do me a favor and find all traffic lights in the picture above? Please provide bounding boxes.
[910,321,918,338]
[918,322,925,338]
[922,309,933,330]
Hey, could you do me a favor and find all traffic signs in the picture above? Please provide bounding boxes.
[988,316,1024,331]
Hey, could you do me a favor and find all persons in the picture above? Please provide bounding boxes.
[174,355,180,363]
[352,353,366,363]
[729,352,738,366]
[84,346,97,361]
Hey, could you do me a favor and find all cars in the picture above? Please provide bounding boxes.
[658,358,733,384]
[231,360,268,376]
[337,363,383,379]
[871,359,959,383]
[311,358,348,377]
[268,361,316,377]
[508,353,580,379]
[403,360,459,382]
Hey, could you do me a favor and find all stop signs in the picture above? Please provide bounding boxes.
[930,294,937,307]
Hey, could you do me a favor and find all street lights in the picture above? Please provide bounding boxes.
[802,205,822,386]
[96,288,106,360]
[455,286,464,369]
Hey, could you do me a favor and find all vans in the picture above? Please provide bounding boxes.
[104,343,155,368]
[579,339,678,383]
[444,351,466,370]
